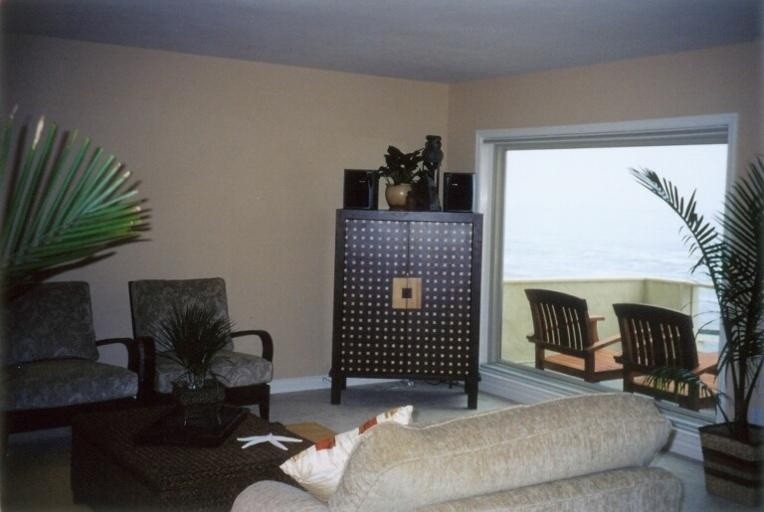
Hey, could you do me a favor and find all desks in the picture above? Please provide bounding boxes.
[64,398,318,511]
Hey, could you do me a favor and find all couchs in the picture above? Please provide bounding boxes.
[220,390,688,512]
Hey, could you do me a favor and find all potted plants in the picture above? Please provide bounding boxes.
[380,142,424,208]
[144,289,235,434]
[627,146,763,512]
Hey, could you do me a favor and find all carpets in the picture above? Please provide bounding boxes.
[283,420,337,443]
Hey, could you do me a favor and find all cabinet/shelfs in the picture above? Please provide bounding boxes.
[326,203,485,411]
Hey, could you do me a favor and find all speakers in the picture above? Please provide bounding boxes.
[443,172,474,212]
[343,169,378,210]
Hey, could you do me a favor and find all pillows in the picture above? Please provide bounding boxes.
[279,402,412,498]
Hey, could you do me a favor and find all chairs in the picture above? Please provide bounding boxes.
[610,302,718,412]
[520,286,626,386]
[1,278,139,447]
[128,276,275,420]
